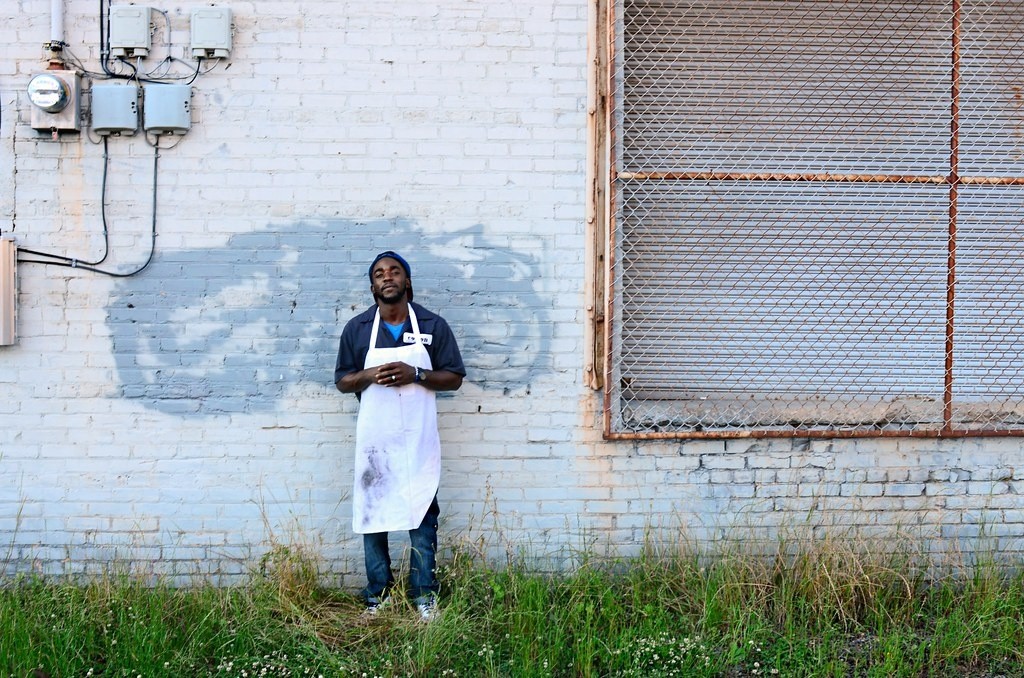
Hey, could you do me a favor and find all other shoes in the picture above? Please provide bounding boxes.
[363,594,392,617]
[418,602,439,620]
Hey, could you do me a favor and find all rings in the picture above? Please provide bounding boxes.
[392,375,396,381]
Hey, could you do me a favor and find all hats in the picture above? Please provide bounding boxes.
[369,251,413,304]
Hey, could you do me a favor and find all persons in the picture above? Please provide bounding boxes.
[334,251,466,618]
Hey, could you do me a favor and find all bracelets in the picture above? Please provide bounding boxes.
[415,366,425,382]
